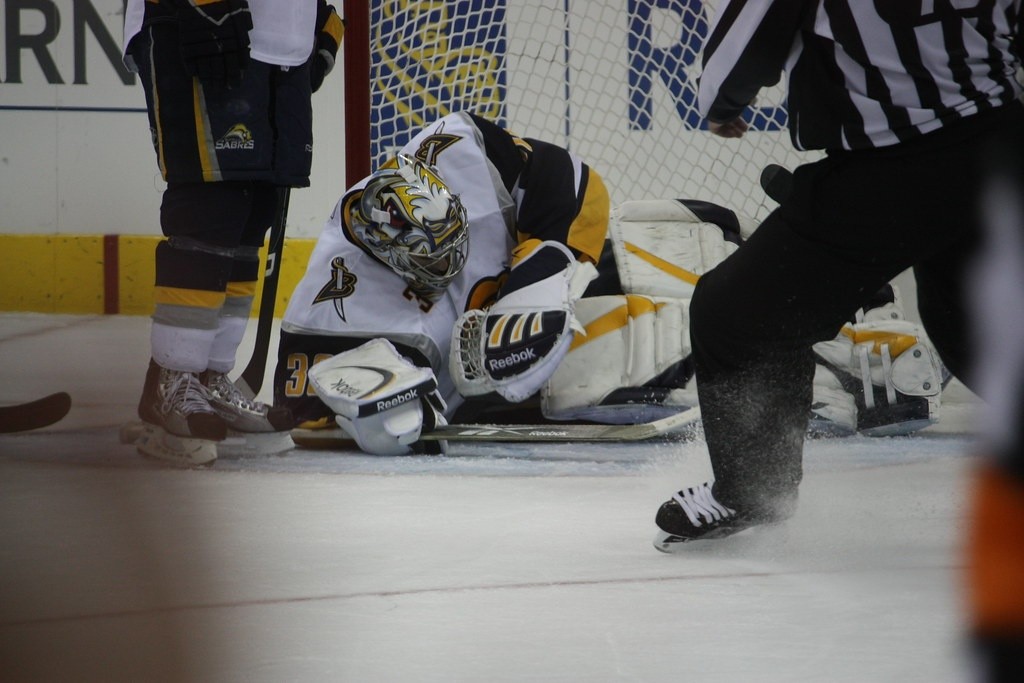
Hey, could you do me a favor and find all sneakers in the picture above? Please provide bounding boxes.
[137,359,226,470]
[190,369,296,456]
[656,480,800,548]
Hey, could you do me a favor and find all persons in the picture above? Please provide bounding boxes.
[264,109,957,456]
[654,1,1024,557]
[118,0,346,464]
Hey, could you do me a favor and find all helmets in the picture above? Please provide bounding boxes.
[352,155,471,302]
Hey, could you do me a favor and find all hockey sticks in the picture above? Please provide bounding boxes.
[287,405,702,447]
[232,189,291,403]
[0,389,74,434]
